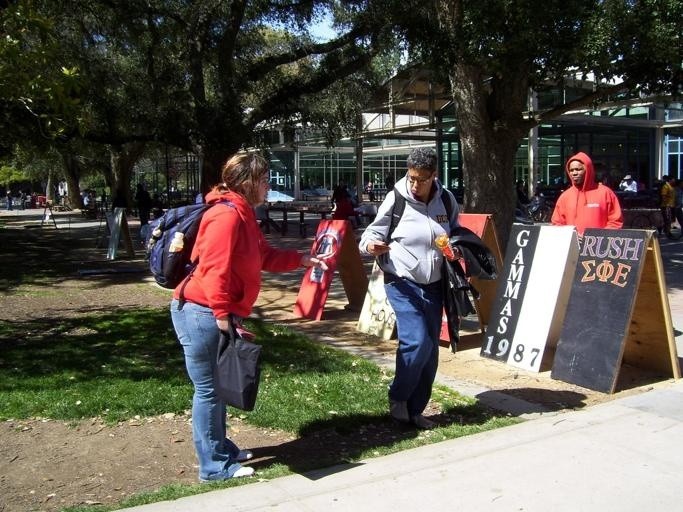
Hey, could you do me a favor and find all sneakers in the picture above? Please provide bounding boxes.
[236,451,252,460]
[388,390,410,430]
[412,414,436,429]
[233,467,254,475]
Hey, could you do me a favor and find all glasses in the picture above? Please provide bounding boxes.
[407,172,433,185]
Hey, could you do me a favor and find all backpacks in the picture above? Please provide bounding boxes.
[141,194,214,290]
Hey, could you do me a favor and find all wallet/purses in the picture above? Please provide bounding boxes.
[235,323,255,342]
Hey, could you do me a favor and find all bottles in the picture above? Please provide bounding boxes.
[168,231,185,252]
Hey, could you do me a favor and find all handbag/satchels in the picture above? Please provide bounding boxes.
[215,314,264,411]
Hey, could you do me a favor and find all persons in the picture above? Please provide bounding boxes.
[100,189,110,206]
[59,179,68,206]
[366,179,377,202]
[171,153,329,481]
[358,147,462,430]
[551,152,623,243]
[135,182,153,227]
[661,174,677,237]
[19,191,27,210]
[330,184,364,228]
[5,190,14,210]
[84,195,98,210]
[619,174,640,192]
[384,173,395,193]
[672,176,683,233]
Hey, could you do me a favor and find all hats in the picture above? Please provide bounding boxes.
[624,174,631,180]
